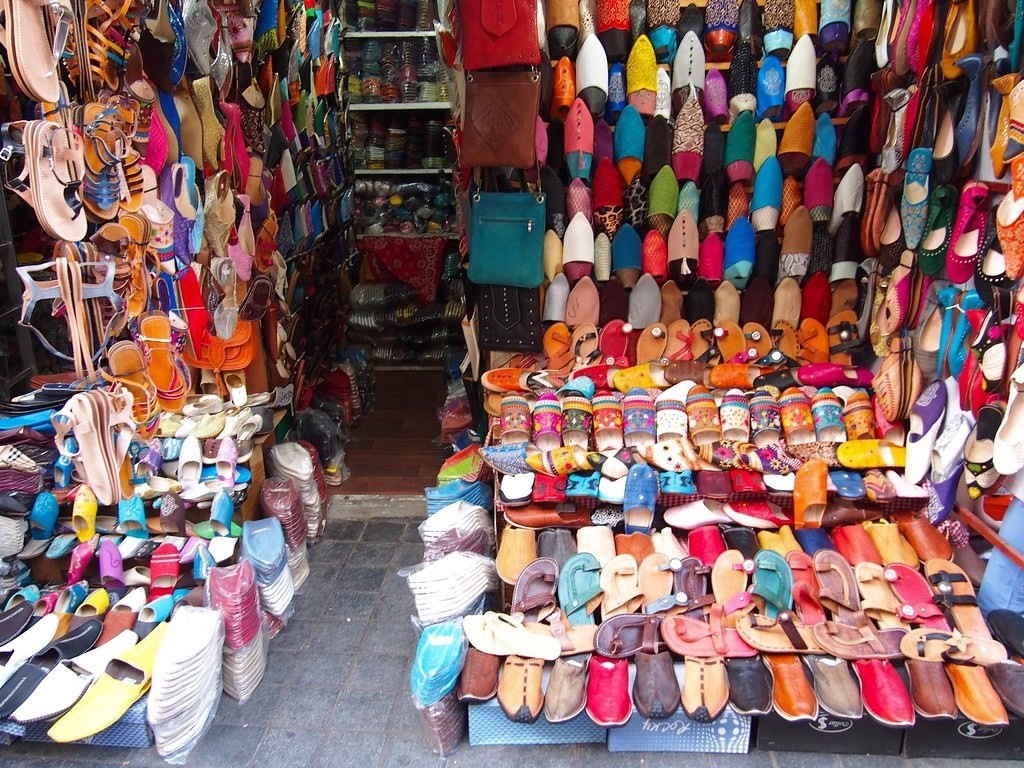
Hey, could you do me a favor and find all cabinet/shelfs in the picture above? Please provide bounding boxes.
[342,32,464,371]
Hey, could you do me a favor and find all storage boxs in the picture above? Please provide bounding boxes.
[466,700,608,745]
[21,691,154,749]
[756,665,905,756]
[899,663,1023,759]
[607,663,752,754]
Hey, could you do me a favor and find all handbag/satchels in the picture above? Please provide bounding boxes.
[477,284,543,352]
[467,163,544,288]
[460,0,540,70]
[461,65,540,168]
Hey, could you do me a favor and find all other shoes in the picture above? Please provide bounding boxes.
[454,0,1024,726]
[0,0,377,741]
